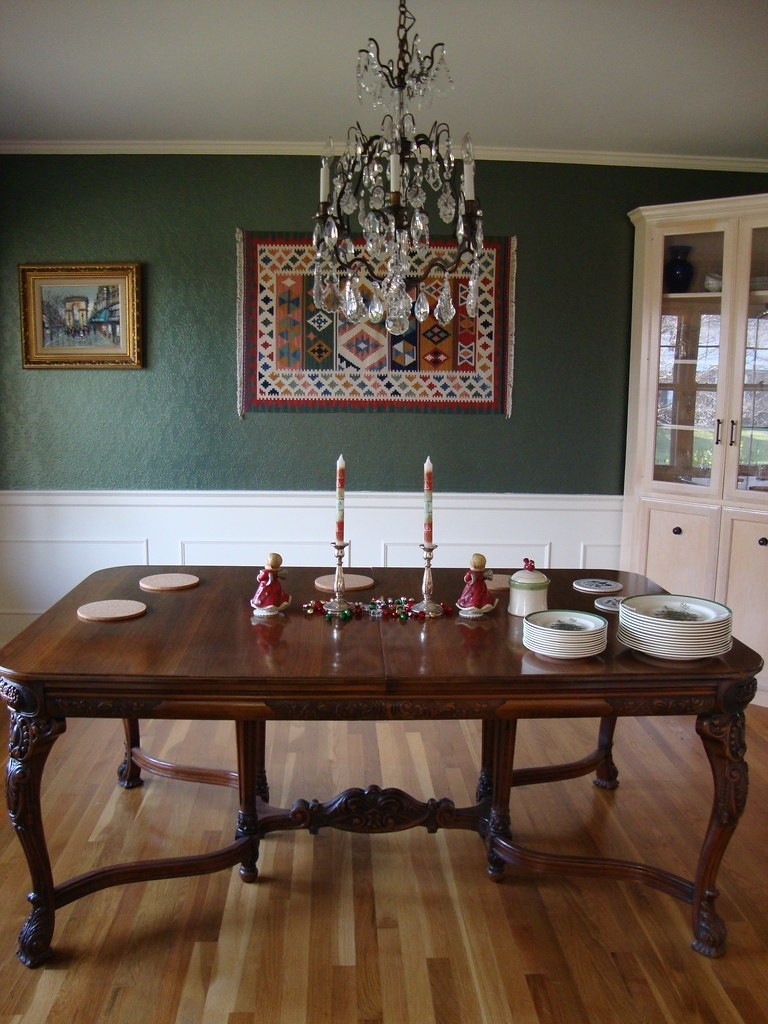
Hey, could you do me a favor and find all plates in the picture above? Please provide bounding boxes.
[615,594,733,660]
[522,610,608,660]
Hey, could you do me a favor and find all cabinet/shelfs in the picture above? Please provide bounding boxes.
[624,194,768,707]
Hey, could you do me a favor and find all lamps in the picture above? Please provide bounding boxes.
[313,0,484,335]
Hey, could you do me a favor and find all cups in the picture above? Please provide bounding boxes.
[507,557,550,617]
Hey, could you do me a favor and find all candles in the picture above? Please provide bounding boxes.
[424,455,433,546]
[335,453,346,545]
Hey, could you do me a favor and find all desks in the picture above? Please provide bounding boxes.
[0,566,764,967]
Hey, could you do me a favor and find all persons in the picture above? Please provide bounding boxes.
[251,553,291,611]
[456,553,500,612]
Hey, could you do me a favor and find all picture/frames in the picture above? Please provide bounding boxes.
[18,265,142,371]
